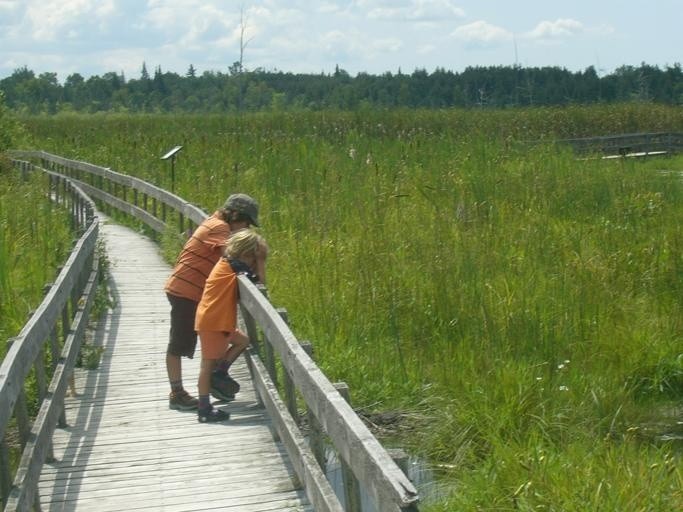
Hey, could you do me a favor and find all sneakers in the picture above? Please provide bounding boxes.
[169,389,198,410]
[197,403,229,423]
[210,369,239,395]
[210,384,235,401]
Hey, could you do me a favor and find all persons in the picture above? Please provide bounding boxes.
[194,226,269,424]
[162,193,260,411]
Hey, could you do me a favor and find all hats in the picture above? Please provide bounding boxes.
[223,194,260,228]
